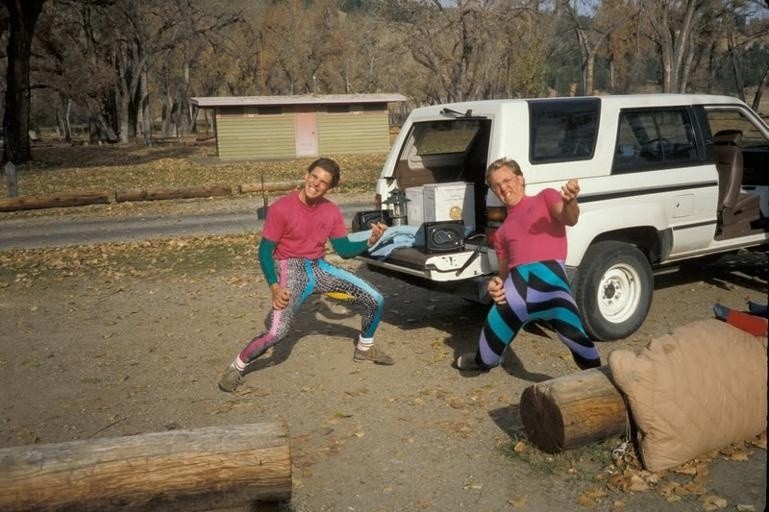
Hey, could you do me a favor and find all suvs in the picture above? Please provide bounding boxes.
[353,92,768,343]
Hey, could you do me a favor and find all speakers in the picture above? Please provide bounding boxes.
[351,209,393,232]
[415,219,465,253]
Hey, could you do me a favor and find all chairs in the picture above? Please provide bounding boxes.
[713,126,763,235]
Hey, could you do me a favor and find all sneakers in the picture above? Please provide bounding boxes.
[352,344,394,366]
[218,365,244,393]
[457,352,482,371]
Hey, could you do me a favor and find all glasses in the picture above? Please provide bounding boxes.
[490,177,517,192]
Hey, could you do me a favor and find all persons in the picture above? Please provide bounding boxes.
[454,156,602,373]
[218,158,396,391]
